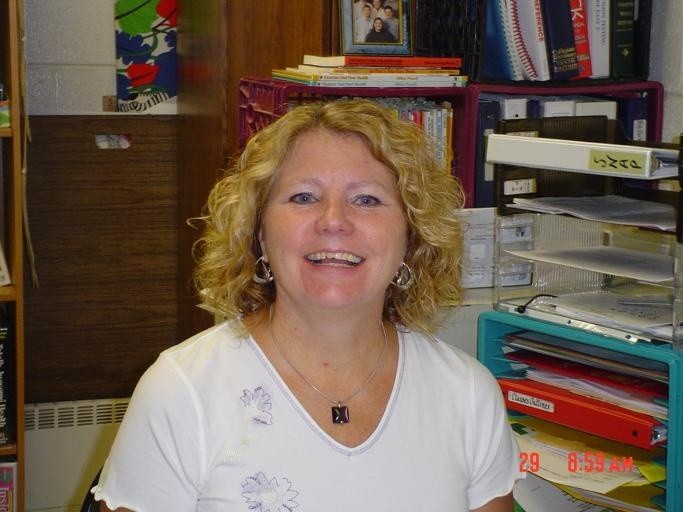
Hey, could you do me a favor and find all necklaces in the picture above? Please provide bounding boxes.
[269,303,387,424]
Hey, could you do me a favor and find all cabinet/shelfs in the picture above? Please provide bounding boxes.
[0,7,25,511]
[464,0,682,511]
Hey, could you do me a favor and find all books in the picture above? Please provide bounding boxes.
[271,54,470,175]
[482,0,635,81]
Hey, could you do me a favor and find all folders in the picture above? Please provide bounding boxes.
[497,329,669,450]
[486,132,681,180]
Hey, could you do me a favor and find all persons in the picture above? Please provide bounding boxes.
[91,100,527,512]
[354,0,398,43]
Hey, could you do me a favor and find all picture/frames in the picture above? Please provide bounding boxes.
[339,0,414,55]
